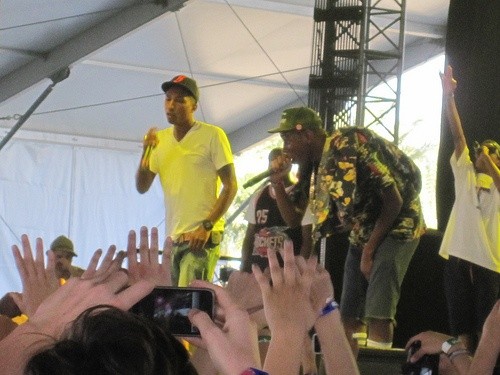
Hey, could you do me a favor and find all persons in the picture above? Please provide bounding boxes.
[268,105,426,349]
[0,225,361,375]
[438,64,500,375]
[405,298,500,374]
[240,147,314,272]
[135,73,238,287]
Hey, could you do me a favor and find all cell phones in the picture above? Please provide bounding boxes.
[122,285,216,337]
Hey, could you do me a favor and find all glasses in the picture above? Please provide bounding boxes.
[488,145,499,158]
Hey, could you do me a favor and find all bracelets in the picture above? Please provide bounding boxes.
[448,348,467,361]
[241,368,269,375]
[321,300,339,316]
[444,93,455,98]
[442,337,462,354]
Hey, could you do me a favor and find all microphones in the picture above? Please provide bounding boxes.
[142,127,156,169]
[242,158,291,189]
[477,145,489,168]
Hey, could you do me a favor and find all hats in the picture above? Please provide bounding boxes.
[45,235,78,258]
[267,106,322,133]
[161,75,199,102]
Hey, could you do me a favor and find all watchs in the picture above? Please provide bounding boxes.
[200,220,214,231]
